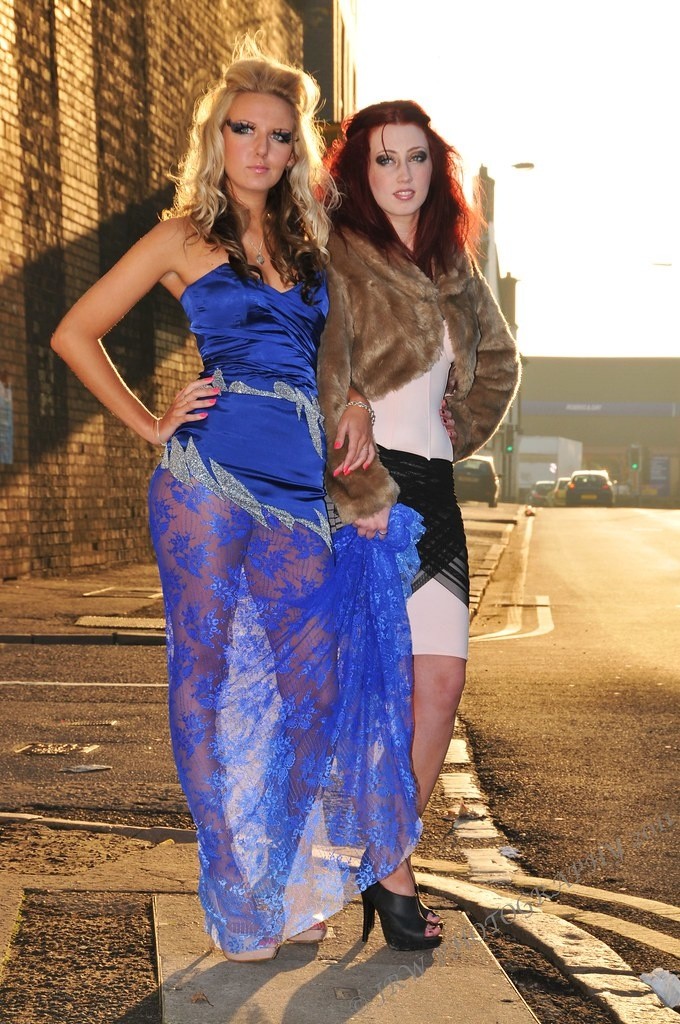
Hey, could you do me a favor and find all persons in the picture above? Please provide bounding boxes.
[51,60,425,960]
[316,100,521,952]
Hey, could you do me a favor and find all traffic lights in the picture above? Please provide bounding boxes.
[631,446,640,469]
[505,425,514,454]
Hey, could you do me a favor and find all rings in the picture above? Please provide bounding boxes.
[362,447,369,451]
[378,531,387,535]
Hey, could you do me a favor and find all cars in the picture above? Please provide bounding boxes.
[566,471,617,506]
[453,456,504,507]
[524,481,555,506]
[546,477,571,507]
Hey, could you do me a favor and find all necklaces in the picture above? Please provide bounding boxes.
[244,234,265,265]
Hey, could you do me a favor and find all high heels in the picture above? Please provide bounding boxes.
[282,907,327,945]
[354,843,443,952]
[205,883,277,963]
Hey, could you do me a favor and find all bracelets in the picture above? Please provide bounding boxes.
[157,420,167,445]
[346,401,375,425]
